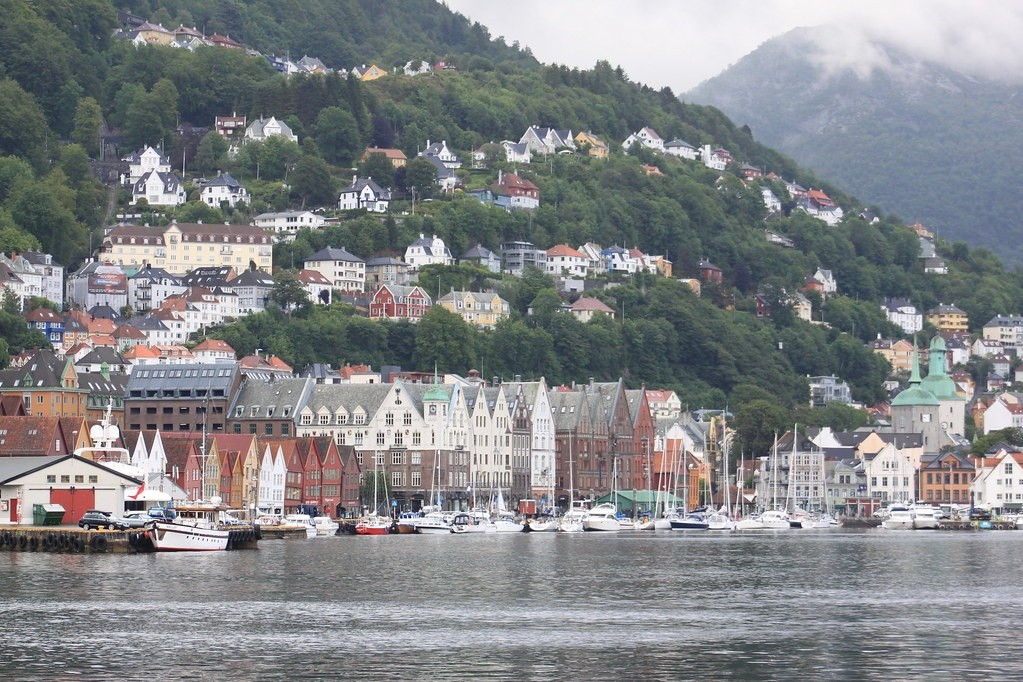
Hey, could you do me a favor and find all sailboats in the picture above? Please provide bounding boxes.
[414,425,1023,535]
[354,413,395,535]
[128,382,263,555]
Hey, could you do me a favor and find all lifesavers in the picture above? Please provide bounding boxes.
[129,534,138,544]
[254,524,262,540]
[229,531,253,542]
[992,524,1014,530]
[939,522,975,529]
[0,532,107,553]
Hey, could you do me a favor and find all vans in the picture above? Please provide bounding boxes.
[873,508,890,517]
[573,500,588,510]
[940,504,951,518]
[421,505,441,518]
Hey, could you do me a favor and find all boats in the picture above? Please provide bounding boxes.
[280,514,317,539]
[314,517,340,538]
[396,512,421,535]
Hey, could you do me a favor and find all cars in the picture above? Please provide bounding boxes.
[955,509,967,520]
[750,512,759,518]
[218,510,239,526]
[676,506,686,515]
[933,509,944,518]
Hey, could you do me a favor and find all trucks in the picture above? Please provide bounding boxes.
[149,506,176,523]
[970,509,991,520]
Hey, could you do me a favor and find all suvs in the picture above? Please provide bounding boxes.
[78,510,130,531]
[120,510,161,528]
